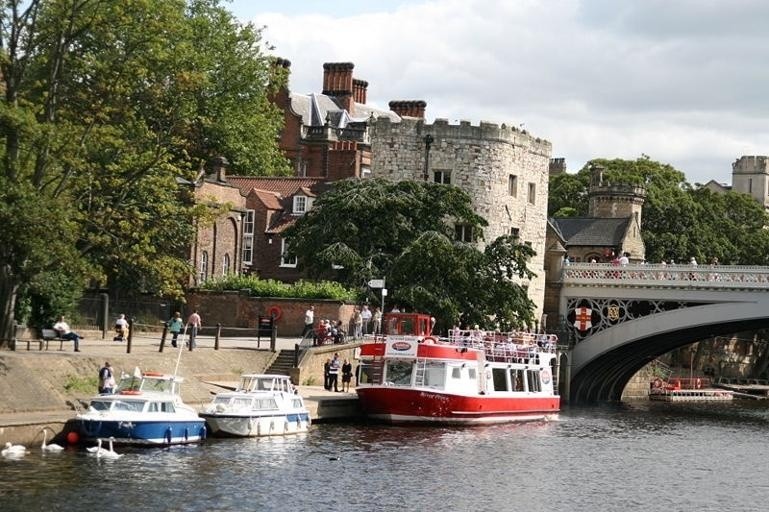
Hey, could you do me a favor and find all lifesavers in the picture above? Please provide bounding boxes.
[268,306,282,321]
[696,378,702,389]
[654,378,663,389]
[119,389,141,395]
[420,338,437,344]
[142,371,163,376]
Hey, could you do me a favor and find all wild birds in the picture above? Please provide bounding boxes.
[329,456,340,460]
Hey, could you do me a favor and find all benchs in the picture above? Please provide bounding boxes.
[14,327,71,350]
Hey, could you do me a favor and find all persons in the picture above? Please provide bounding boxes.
[564,255,571,267]
[186,308,202,348]
[611,253,719,266]
[53,314,84,351]
[452,319,548,357]
[590,256,597,263]
[301,301,400,346]
[116,313,129,340]
[97,361,113,393]
[166,311,184,347]
[324,352,353,393]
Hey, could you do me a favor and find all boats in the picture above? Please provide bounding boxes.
[68,372,314,444]
[350,326,566,421]
[648,378,769,403]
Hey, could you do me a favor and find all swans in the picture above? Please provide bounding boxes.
[85,436,116,453]
[1,442,26,456]
[96,438,124,460]
[41,429,65,451]
